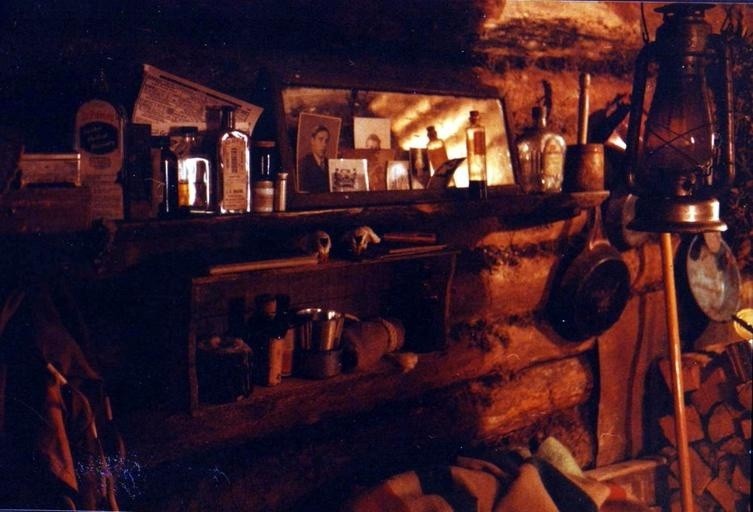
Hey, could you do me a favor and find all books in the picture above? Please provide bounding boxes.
[205,252,318,275]
[387,236,450,255]
[381,231,436,244]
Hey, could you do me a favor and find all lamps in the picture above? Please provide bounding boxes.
[620,0,736,234]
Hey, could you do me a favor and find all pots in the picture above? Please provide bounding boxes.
[551,204,631,342]
[677,229,744,322]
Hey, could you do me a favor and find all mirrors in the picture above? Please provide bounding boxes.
[273,75,526,212]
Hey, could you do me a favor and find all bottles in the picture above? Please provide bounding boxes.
[174,126,217,218]
[250,294,296,389]
[211,104,254,217]
[466,110,488,200]
[427,125,456,188]
[252,138,289,213]
[515,104,567,195]
[149,134,178,220]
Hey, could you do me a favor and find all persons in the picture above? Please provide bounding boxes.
[332,166,362,193]
[299,126,330,194]
[364,134,380,149]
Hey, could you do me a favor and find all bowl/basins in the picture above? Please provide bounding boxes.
[296,308,349,354]
